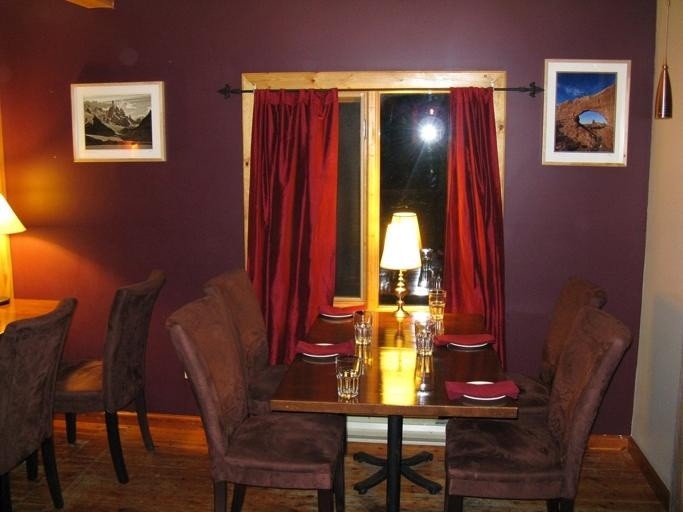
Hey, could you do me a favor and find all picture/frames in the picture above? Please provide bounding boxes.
[68,80,168,164]
[540,57,633,170]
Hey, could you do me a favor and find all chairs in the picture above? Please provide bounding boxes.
[162,295,346,512]
[26,269,172,486]
[0,295,79,512]
[439,273,610,419]
[437,304,635,511]
[201,265,351,458]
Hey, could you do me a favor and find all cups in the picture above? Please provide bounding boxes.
[334,356,360,399]
[414,320,434,355]
[428,289,447,320]
[353,310,372,345]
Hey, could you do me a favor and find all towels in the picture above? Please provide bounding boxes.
[317,304,367,318]
[294,338,356,358]
[443,378,520,402]
[433,330,495,349]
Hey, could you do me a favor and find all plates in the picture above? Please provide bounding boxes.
[321,312,352,318]
[303,343,338,358]
[449,340,489,349]
[461,380,506,402]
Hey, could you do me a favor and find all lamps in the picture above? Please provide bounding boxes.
[391,213,422,253]
[0,193,28,306]
[379,224,423,319]
[654,5,673,119]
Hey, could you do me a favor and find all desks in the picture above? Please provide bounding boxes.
[0,299,59,336]
